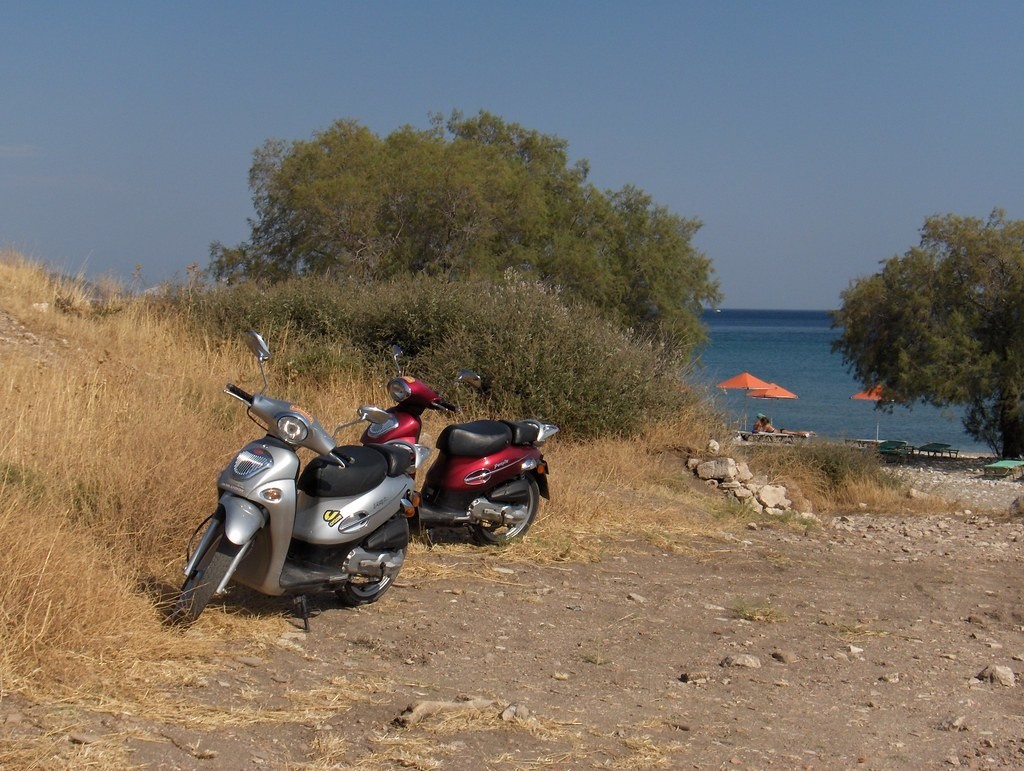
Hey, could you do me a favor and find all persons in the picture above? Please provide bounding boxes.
[759,418,806,438]
[753,412,772,433]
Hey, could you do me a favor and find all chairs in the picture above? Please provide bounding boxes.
[983,460,1024,482]
[739,429,813,443]
[877,438,961,463]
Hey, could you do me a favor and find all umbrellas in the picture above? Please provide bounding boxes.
[746,382,799,430]
[716,371,776,430]
[849,382,905,440]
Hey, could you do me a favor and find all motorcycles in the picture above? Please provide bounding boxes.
[361,344,559,547]
[165,326,431,635]
